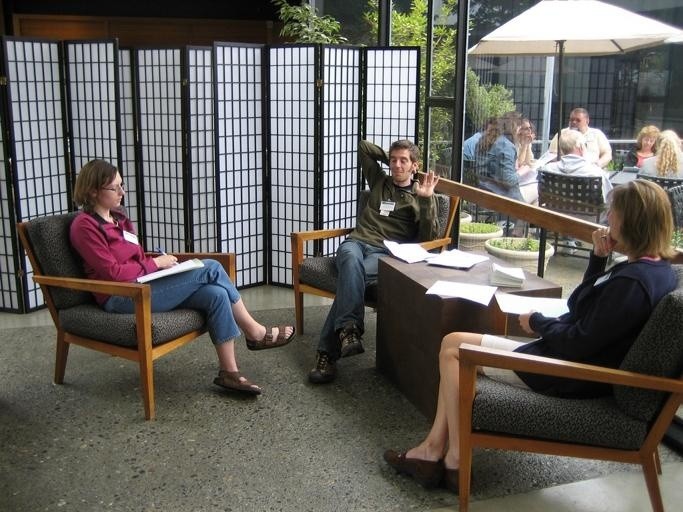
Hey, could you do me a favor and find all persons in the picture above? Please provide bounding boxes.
[69,159,296,395]
[309,139,440,384]
[384,178,678,494]
[462,107,683,262]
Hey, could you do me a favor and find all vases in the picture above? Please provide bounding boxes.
[459,210,472,224]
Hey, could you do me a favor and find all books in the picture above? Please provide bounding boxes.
[487,263,525,288]
[136,258,204,284]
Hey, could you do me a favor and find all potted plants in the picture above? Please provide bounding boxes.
[458,221,503,249]
[483,236,555,274]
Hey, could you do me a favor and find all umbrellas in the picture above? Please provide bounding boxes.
[467,0,683,162]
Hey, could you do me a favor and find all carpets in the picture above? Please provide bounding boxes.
[2,303,682,511]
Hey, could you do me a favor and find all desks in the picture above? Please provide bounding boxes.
[606,168,642,187]
[373,250,564,425]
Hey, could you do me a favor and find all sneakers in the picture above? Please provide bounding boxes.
[443,459,474,495]
[561,241,579,258]
[338,326,365,358]
[308,350,337,385]
[383,448,444,492]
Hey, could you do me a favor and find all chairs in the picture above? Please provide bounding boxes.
[14,205,238,420]
[462,160,513,233]
[454,264,682,511]
[665,183,683,226]
[636,173,683,191]
[536,170,615,279]
[290,189,462,338]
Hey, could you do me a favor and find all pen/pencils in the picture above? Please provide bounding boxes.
[157,248,180,267]
[599,234,607,240]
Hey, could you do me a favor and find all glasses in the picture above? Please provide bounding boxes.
[94,182,126,193]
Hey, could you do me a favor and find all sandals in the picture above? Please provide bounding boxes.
[213,369,262,399]
[244,324,297,350]
